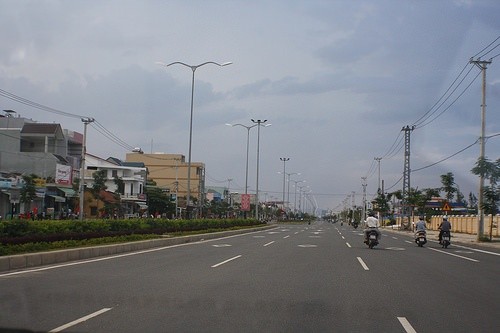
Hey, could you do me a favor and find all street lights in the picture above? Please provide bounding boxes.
[226,122,271,220]
[166,60,233,220]
[277,170,319,220]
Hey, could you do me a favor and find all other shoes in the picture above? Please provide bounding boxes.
[359,232,452,245]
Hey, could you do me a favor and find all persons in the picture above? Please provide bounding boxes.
[437,215,452,244]
[413,216,427,242]
[327,217,359,229]
[18,204,160,221]
[363,212,382,244]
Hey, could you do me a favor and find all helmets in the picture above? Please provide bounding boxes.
[443,215,448,219]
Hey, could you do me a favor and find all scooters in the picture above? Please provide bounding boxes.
[363,229,379,249]
[415,226,427,246]
[437,223,452,249]
